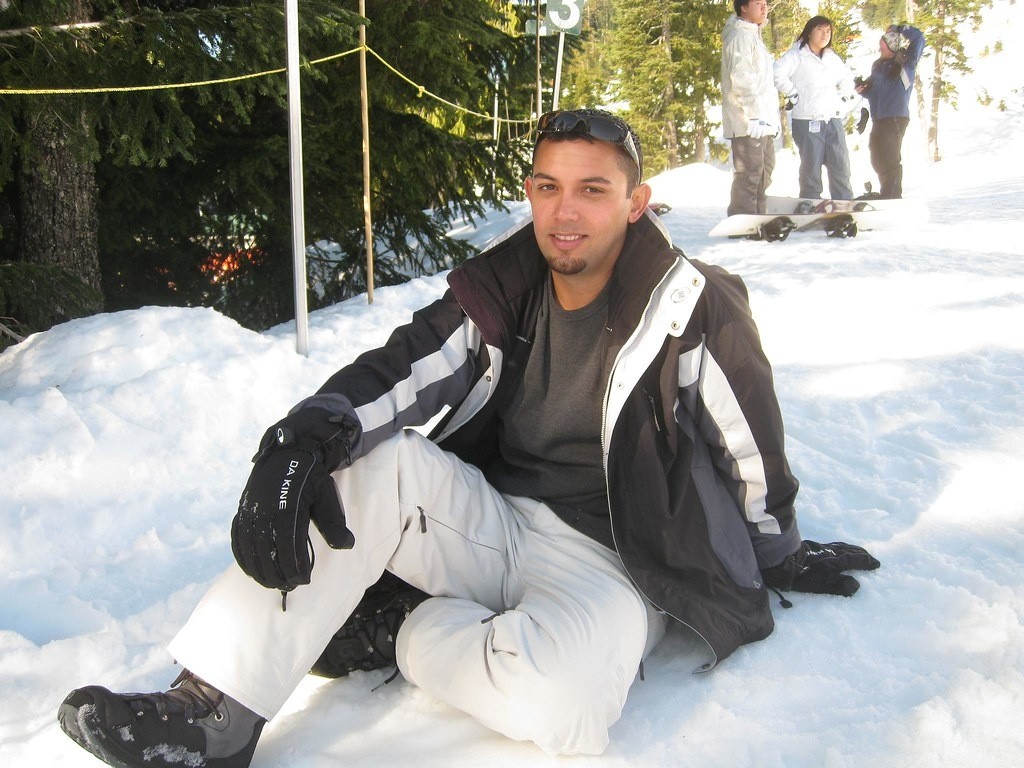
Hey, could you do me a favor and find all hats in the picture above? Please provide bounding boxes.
[883,31,901,52]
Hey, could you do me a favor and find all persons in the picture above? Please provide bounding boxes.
[57,105,880,768]
[774,15,870,198]
[722,0,782,240]
[853,21,925,199]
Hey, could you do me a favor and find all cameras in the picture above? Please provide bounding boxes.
[855,77,871,92]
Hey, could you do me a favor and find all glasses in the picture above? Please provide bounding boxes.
[536,111,642,186]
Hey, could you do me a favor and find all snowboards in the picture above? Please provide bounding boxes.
[706,210,882,243]
[726,194,906,217]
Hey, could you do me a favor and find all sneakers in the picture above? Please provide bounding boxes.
[56,665,267,768]
[307,585,434,691]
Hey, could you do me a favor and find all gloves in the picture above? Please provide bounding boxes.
[856,108,869,135]
[231,405,355,592]
[747,119,766,140]
[784,89,799,111]
[761,539,881,596]
[773,122,782,140]
